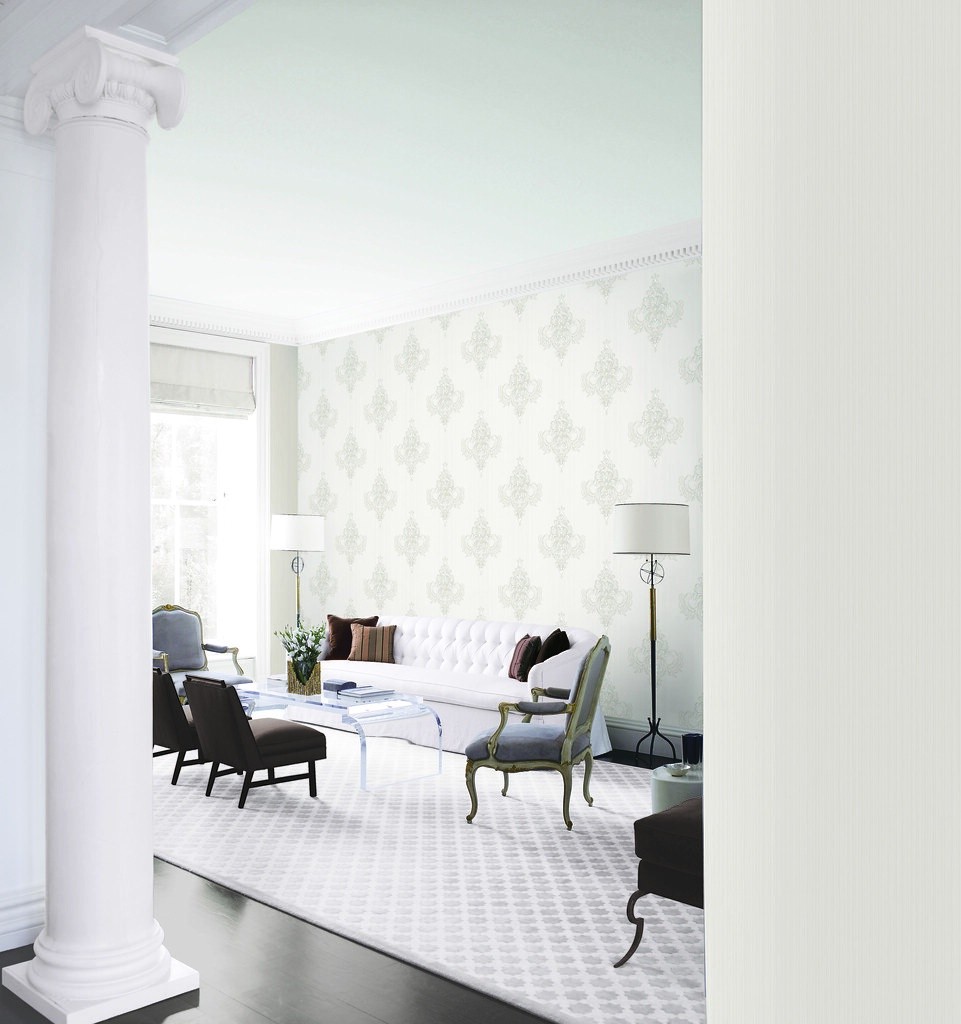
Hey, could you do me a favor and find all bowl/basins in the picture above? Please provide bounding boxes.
[664,763,691,777]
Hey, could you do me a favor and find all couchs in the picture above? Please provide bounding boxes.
[286,615,612,757]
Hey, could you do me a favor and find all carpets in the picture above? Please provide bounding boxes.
[154,690,707,1024]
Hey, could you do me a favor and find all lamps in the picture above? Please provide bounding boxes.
[612,503,690,762]
[269,514,325,631]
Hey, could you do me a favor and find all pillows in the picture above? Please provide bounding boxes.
[324,614,378,661]
[347,623,397,664]
[536,628,571,664]
[508,633,543,683]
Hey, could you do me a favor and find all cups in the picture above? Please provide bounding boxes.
[680,733,703,770]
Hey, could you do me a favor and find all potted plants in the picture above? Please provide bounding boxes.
[273,618,327,694]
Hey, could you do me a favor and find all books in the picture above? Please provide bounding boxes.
[337,686,395,697]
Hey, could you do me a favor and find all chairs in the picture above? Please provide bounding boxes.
[465,636,612,831]
[152,604,256,716]
[613,796,704,969]
[152,666,244,785]
[182,674,327,809]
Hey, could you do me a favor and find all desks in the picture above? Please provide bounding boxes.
[650,763,703,813]
[233,678,443,790]
